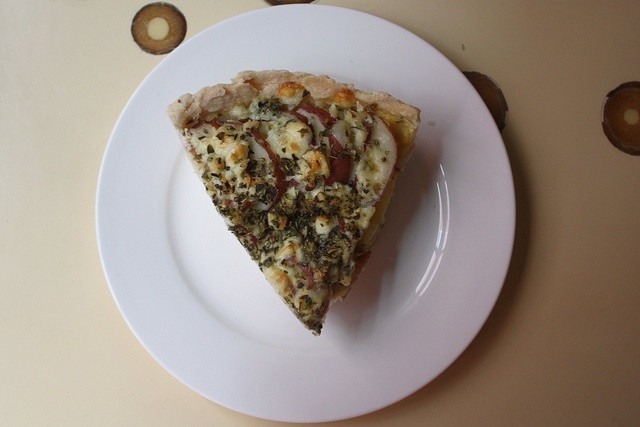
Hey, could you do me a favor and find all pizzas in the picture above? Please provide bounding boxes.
[167,68,421,336]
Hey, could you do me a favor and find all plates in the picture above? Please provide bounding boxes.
[93,3,518,425]
